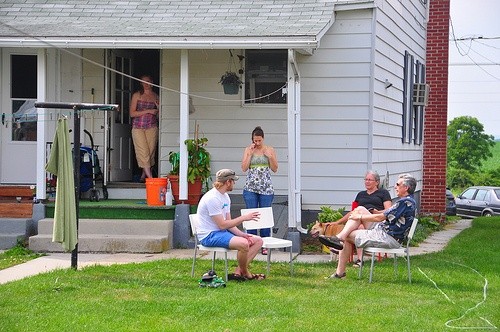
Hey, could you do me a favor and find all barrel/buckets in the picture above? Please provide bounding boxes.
[145,178,167,206]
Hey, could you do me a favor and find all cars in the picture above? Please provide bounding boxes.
[446,186,456,216]
[454,186,500,219]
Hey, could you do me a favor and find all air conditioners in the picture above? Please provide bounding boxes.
[413,83,430,106]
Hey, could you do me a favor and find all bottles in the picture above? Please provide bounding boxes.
[165,189,172,206]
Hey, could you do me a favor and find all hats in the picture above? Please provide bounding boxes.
[216,168,239,182]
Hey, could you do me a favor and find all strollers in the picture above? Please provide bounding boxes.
[67,128,109,202]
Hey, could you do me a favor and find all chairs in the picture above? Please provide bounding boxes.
[358,217,418,284]
[189,214,229,283]
[241,206,293,277]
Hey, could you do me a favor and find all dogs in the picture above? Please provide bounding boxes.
[308,219,345,264]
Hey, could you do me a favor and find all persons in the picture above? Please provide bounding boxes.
[241,126,278,255]
[129,74,159,183]
[325,172,392,268]
[319,173,417,280]
[196,169,266,281]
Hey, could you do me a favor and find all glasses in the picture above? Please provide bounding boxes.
[364,178,377,181]
[396,183,400,186]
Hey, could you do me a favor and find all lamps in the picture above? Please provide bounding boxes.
[386,79,393,88]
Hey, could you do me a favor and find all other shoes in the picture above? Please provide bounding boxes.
[262,248,268,255]
[140,175,146,183]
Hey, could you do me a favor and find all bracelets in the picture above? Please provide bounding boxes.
[359,214,362,220]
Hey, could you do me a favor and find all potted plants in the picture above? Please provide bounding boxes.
[306,206,346,253]
[167,137,212,205]
[218,72,242,95]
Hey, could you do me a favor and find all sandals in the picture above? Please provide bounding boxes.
[249,273,265,280]
[352,259,364,268]
[325,271,346,280]
[325,244,339,254]
[233,273,248,280]
[318,234,345,250]
